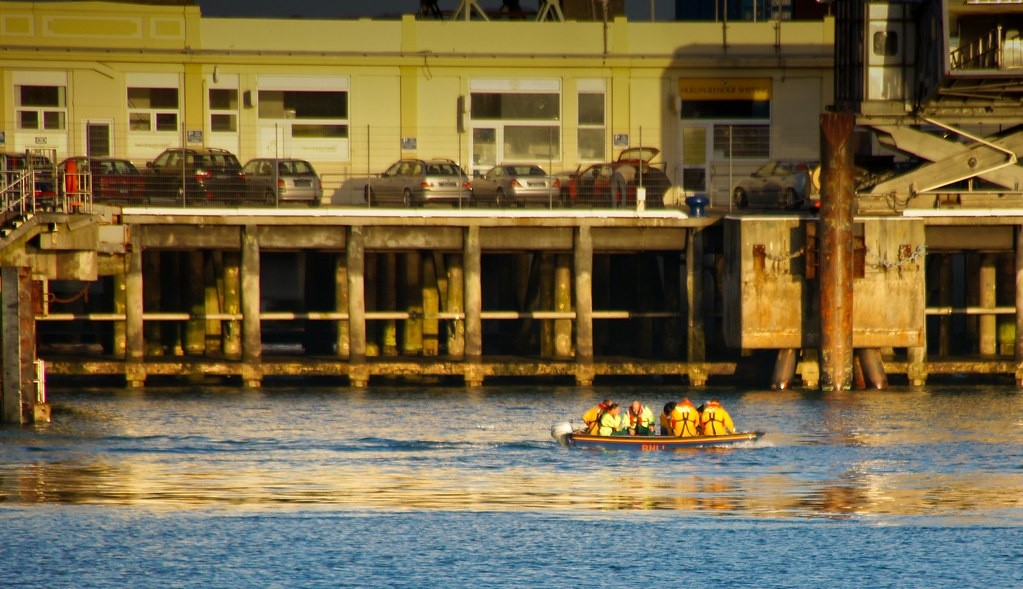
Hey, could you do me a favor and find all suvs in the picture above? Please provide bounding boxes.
[364,157,474,207]
[139,148,246,207]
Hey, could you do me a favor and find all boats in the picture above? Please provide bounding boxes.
[551,423,765,451]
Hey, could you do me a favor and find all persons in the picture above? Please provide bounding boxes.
[582,396,740,436]
[610,163,639,208]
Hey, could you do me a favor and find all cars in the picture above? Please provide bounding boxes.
[243,159,323,207]
[470,163,560,206]
[0,153,59,197]
[57,156,145,204]
[735,158,820,212]
[559,147,671,207]
